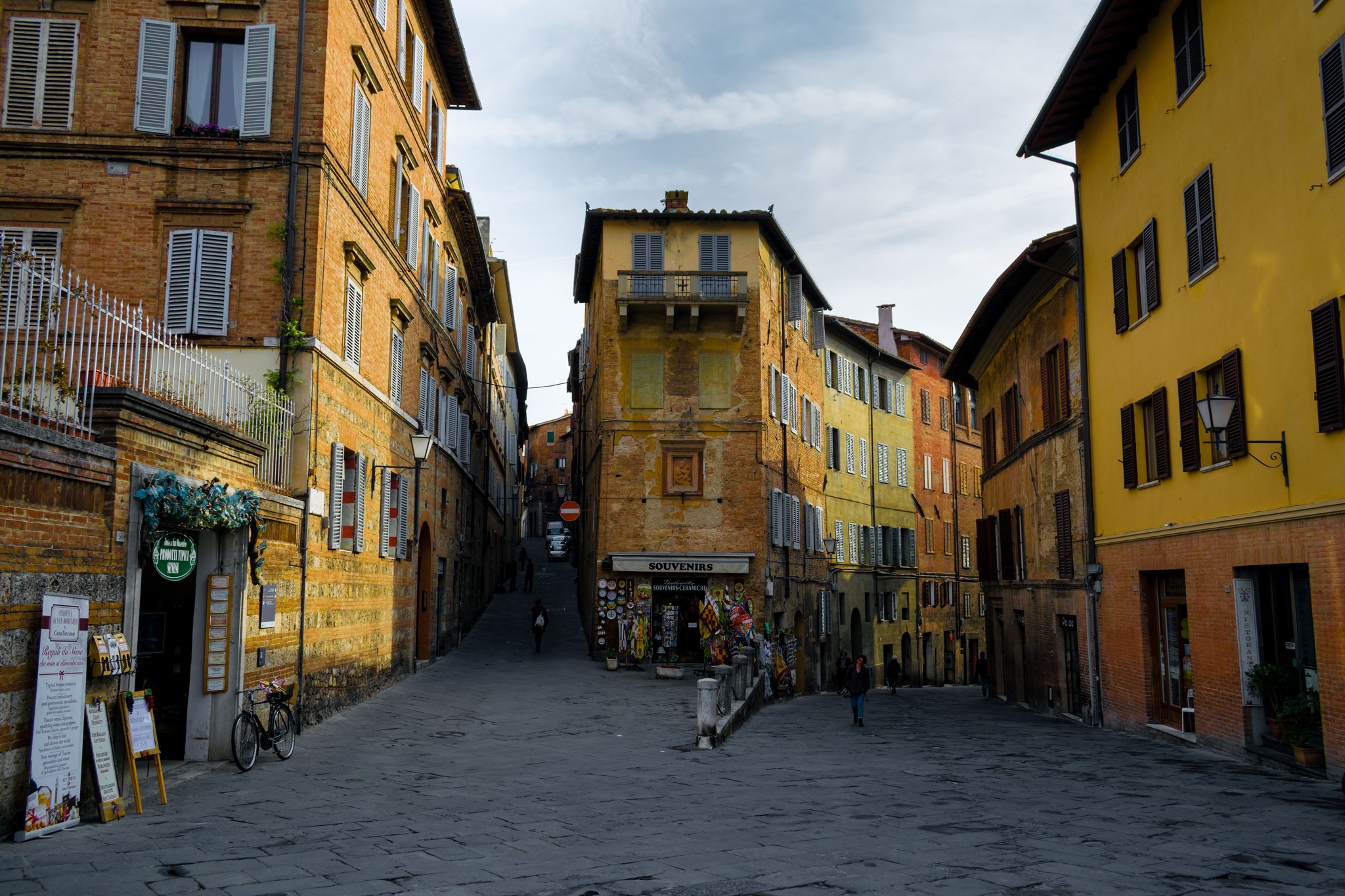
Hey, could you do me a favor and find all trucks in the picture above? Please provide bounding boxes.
[545,521,565,549]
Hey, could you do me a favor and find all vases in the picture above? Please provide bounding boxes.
[80,369,120,387]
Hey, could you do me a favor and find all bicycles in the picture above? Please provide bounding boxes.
[231,680,296,771]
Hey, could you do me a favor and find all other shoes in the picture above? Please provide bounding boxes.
[859,721,864,727]
[854,716,858,724]
[837,691,842,695]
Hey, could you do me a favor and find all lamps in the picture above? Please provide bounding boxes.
[372,434,436,498]
[555,478,587,504]
[1195,382,1289,488]
[497,484,532,508]
[656,647,666,657]
[803,533,838,578]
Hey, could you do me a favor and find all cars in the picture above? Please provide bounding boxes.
[547,541,569,554]
[564,528,569,531]
[551,536,570,548]
[564,530,572,541]
[548,544,569,562]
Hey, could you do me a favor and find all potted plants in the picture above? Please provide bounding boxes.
[1,381,97,442]
[603,647,618,659]
[660,655,680,669]
[1244,662,1325,768]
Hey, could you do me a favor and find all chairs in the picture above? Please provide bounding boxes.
[1182,689,1194,733]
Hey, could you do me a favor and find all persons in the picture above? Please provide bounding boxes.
[846,654,870,727]
[531,600,548,652]
[522,559,534,592]
[977,652,988,697]
[509,558,518,591]
[887,655,902,694]
[835,650,852,696]
[519,547,528,570]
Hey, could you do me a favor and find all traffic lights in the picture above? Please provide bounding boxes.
[949,631,954,641]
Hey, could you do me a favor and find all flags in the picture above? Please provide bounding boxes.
[699,582,756,666]
[776,645,789,685]
[618,612,653,660]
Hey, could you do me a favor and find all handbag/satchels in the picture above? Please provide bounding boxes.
[886,681,892,691]
[842,687,850,699]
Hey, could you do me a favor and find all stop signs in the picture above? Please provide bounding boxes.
[560,501,580,521]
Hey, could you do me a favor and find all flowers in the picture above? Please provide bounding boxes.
[175,123,240,138]
[76,352,209,417]
